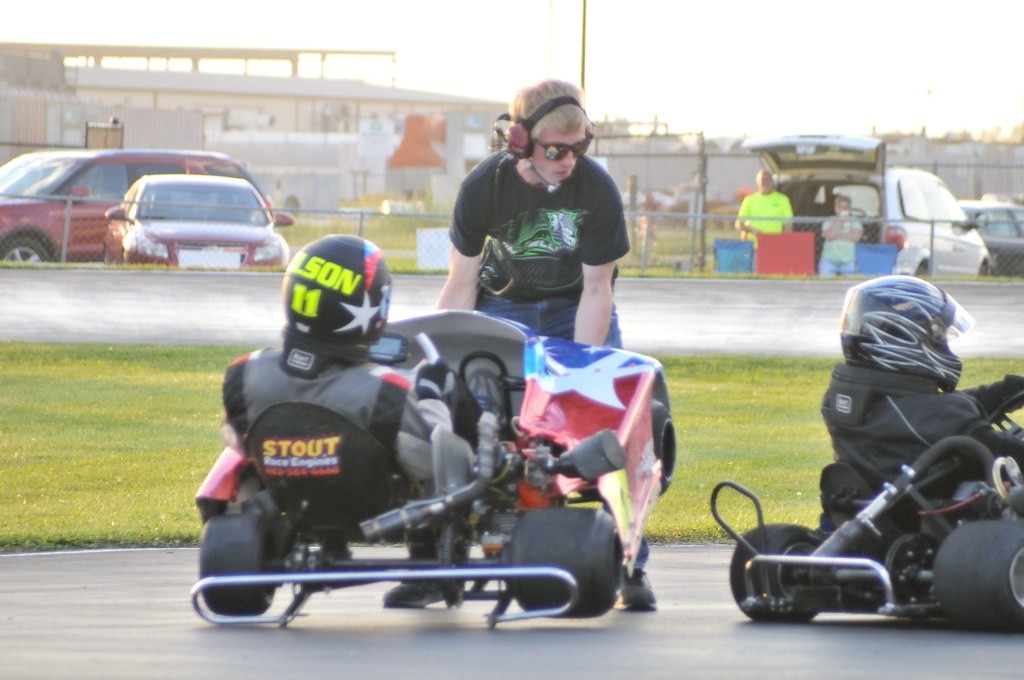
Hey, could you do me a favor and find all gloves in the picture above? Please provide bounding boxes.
[999,374,1024,413]
[415,357,457,401]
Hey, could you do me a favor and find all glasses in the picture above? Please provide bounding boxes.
[531,132,595,161]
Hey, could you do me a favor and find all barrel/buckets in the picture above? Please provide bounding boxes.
[415,228,450,269]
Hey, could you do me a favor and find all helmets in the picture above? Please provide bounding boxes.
[840,275,963,391]
[281,234,390,344]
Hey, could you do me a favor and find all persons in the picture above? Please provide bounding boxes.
[219,228,510,548]
[734,167,865,279]
[382,74,662,613]
[818,271,1024,536]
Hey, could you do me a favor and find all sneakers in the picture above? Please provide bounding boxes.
[466,368,508,432]
[383,576,464,608]
[610,564,655,610]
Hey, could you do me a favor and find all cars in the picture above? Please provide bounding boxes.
[959,199,1024,278]
[102,174,297,273]
[0,149,273,264]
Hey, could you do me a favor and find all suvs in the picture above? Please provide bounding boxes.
[741,134,991,279]
